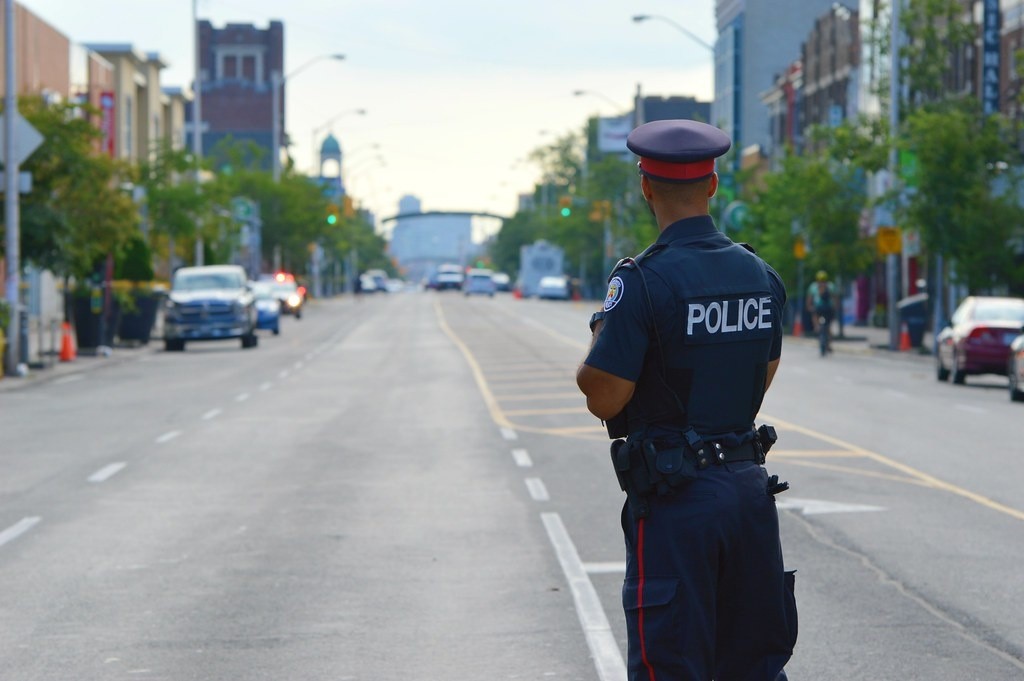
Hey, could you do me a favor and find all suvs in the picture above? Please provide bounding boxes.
[161,264,255,351]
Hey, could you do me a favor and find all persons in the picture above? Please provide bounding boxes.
[576,119,799,680]
[807,270,839,351]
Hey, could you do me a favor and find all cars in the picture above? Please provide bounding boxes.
[250,274,306,334]
[461,268,497,297]
[936,294,1024,402]
[539,276,570,301]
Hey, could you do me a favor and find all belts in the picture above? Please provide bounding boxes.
[686,441,759,466]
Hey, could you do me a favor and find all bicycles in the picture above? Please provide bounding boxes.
[813,304,834,359]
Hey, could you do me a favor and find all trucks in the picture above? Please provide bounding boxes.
[436,263,463,287]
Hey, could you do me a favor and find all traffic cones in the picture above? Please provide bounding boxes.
[899,323,912,350]
[793,311,803,337]
[515,285,521,297]
[572,287,579,301]
[57,323,76,363]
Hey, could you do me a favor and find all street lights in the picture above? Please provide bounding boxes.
[268,53,347,271]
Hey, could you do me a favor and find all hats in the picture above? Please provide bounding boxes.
[625,120,731,184]
[815,270,828,282]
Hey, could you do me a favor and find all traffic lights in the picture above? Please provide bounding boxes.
[324,206,338,231]
[560,194,574,219]
